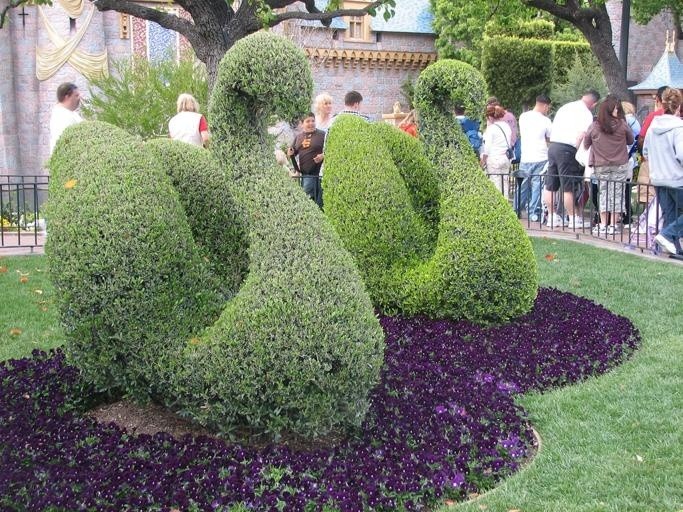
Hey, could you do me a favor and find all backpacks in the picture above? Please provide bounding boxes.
[461,125,481,149]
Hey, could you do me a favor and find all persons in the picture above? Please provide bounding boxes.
[168,92,210,150]
[268,91,373,209]
[453,86,683,256]
[48,83,84,157]
[398,108,418,138]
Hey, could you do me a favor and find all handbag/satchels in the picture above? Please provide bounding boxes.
[506,139,521,164]
[575,184,590,212]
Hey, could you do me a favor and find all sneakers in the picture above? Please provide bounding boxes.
[546,213,621,235]
[654,233,677,255]
